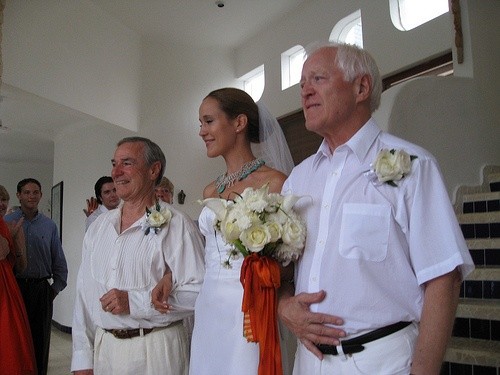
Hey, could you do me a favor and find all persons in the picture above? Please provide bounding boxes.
[70,136,205,375]
[189,88,288,374]
[277,41,476,375]
[1,178,68,374]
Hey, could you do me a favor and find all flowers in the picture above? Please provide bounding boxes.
[195,182,313,267]
[145,194,173,236]
[371,148,419,187]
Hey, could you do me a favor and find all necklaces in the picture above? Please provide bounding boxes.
[215,159,266,194]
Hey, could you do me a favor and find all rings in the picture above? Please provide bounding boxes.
[106,306,111,312]
[150,303,155,308]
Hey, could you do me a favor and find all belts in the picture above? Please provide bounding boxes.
[102,327,154,340]
[312,321,414,355]
